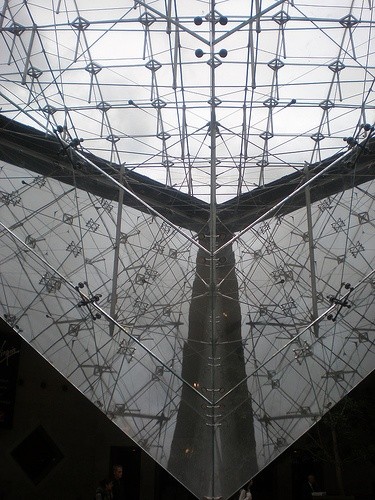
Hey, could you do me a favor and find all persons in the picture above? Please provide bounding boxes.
[104,465,125,500]
[91,475,114,500]
[238,479,254,500]
[301,474,319,499]
